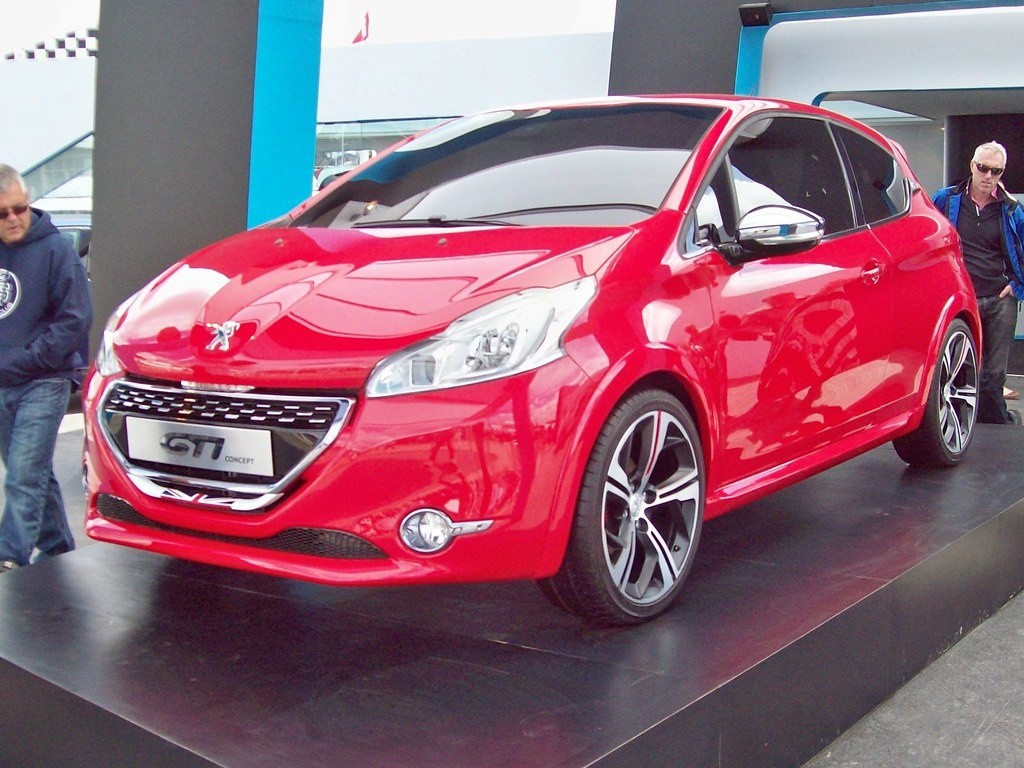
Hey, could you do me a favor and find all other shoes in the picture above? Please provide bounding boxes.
[1008,409,1022,425]
[0,560,19,575]
[1002,390,1019,398]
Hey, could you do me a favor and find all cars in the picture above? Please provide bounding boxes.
[72,89,984,626]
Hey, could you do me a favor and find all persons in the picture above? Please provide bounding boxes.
[931,142,1024,425]
[0,165,91,573]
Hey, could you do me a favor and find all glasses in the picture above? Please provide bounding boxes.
[974,161,1004,175]
[0,204,28,219]
